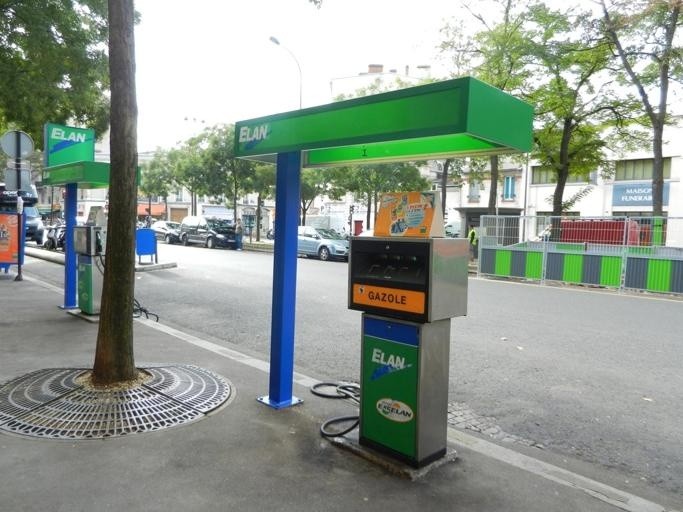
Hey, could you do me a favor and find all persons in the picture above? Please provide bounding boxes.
[467,224,477,262]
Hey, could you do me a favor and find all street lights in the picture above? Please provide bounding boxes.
[270,36,301,108]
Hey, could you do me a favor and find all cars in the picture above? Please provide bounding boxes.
[151,216,238,250]
[24,207,44,244]
[297,226,349,261]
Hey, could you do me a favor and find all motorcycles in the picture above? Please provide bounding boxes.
[46,227,65,251]
[343,227,349,238]
